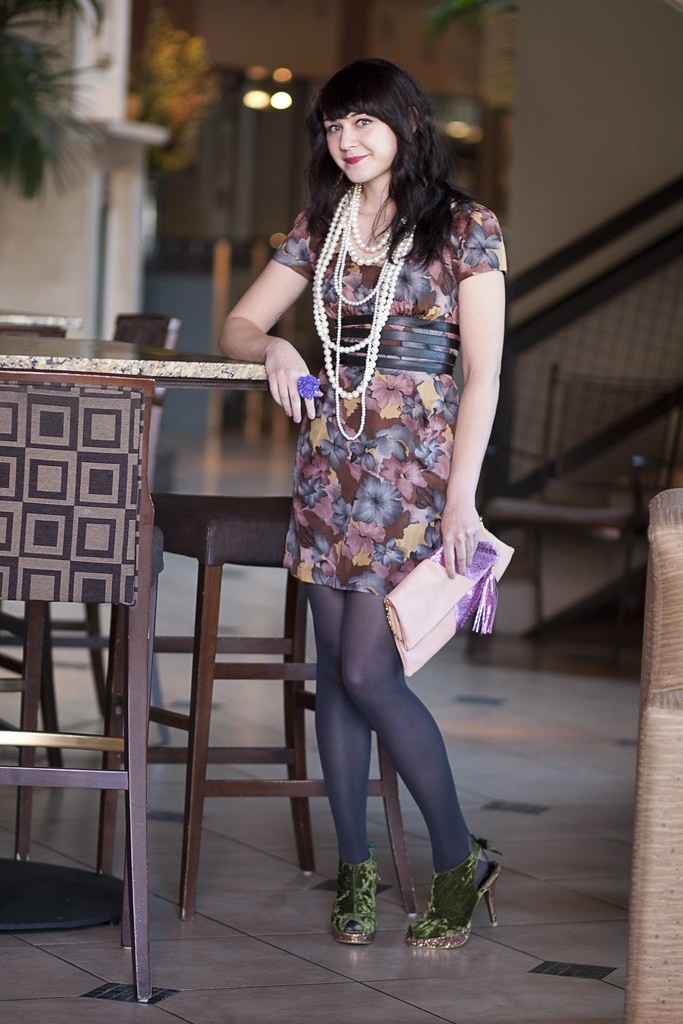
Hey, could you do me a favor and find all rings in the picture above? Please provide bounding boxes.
[296,374,320,401]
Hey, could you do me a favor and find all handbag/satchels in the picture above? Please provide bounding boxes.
[383,516,516,677]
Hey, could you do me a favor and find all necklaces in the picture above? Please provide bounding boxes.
[312,181,416,441]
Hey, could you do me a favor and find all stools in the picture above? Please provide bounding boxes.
[146,492,421,923]
[1,367,153,1005]
[112,313,181,490]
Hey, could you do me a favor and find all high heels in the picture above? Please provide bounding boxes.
[404,835,503,949]
[331,850,379,944]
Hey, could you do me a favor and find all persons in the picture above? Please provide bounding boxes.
[218,62,509,950]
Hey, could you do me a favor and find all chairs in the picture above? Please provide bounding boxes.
[466,366,678,672]
